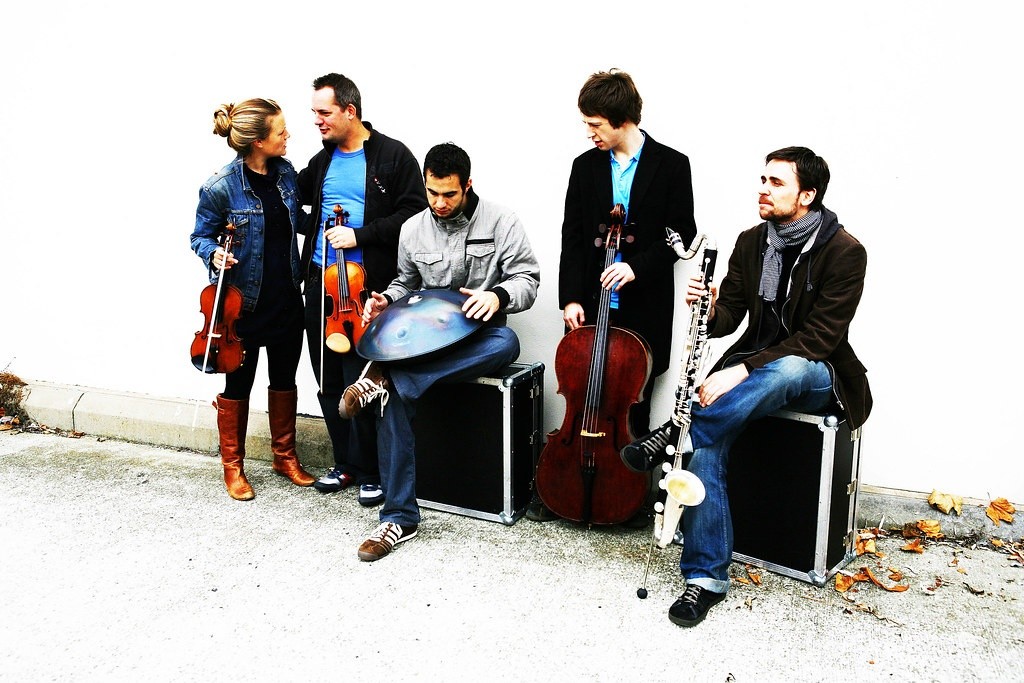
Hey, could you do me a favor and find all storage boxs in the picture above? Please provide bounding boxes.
[415,360,545,523]
[671,390,863,586]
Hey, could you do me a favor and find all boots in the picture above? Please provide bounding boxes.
[266,383,316,486]
[211,392,255,501]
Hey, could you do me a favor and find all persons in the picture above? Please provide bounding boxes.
[523,67,697,526]
[291,70,433,509]
[337,141,543,560]
[612,141,876,630]
[186,93,318,501]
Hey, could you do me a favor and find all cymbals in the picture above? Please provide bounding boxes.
[356,288,487,362]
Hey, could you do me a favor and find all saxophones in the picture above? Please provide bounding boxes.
[654,227,719,551]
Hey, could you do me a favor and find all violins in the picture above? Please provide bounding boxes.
[191,222,246,374]
[324,204,370,354]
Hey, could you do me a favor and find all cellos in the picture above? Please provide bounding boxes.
[535,204,652,526]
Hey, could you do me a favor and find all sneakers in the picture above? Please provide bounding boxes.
[358,478,384,507]
[338,359,392,419]
[667,582,726,624]
[357,519,418,561]
[313,466,352,493]
[619,417,683,475]
